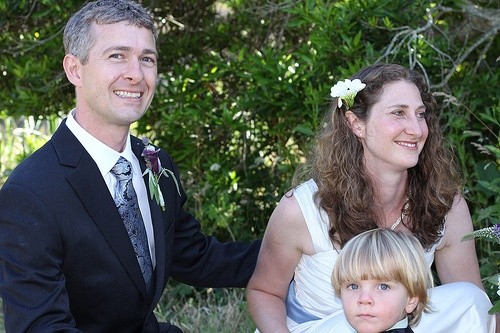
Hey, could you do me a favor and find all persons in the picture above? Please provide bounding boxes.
[242,61,500,333]
[0,1,295,332]
[331,225,437,333]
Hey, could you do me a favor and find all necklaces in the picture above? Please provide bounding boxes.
[389,185,411,230]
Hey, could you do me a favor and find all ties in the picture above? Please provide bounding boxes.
[114,157,154,295]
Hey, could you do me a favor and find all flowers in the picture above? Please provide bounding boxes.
[330,78,365,110]
[139,136,182,211]
